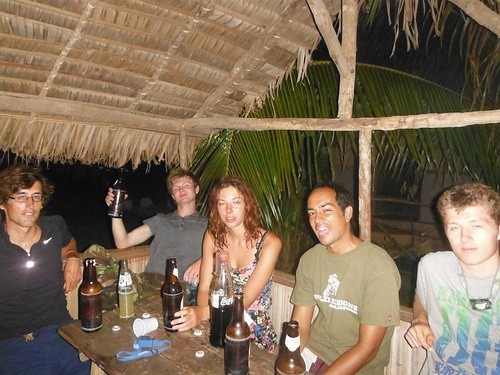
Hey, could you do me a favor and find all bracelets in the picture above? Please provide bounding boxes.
[65,250,78,258]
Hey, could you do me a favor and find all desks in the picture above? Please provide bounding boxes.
[57,281,314,375]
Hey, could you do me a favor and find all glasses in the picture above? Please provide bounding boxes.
[8,193,44,202]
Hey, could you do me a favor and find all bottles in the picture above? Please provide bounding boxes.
[162,258,184,333]
[107,168,127,218]
[78,258,103,333]
[276,321,306,375]
[118,260,135,318]
[223,292,251,375]
[209,251,234,348]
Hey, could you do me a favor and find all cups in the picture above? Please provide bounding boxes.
[133,317,159,337]
[214,252,233,276]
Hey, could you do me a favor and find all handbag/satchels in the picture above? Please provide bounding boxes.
[82,244,144,310]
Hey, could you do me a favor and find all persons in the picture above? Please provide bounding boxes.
[170,177,282,353]
[0,163,93,375]
[284,182,401,375]
[105,166,215,299]
[408,181,500,375]
